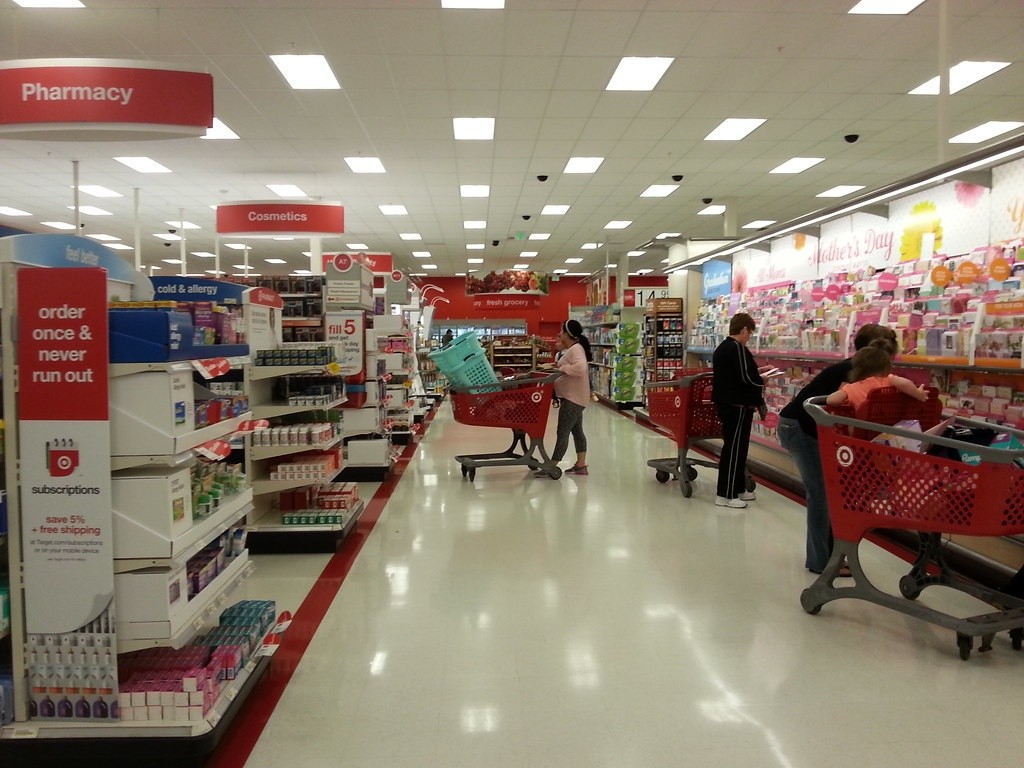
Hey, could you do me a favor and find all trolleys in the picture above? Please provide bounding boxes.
[445,368,564,481]
[799,394,1024,661]
[645,371,757,498]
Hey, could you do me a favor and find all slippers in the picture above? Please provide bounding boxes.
[808,559,851,578]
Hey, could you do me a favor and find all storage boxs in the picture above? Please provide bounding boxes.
[108,300,276,721]
[255,346,360,525]
[751,247,1024,465]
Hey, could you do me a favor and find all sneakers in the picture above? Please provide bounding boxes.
[565,463,590,475]
[534,469,549,478]
[715,494,748,508]
[737,489,755,500]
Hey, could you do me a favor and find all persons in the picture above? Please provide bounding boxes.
[532,320,597,479]
[442,329,453,346]
[710,312,774,507]
[827,338,929,412]
[778,323,898,575]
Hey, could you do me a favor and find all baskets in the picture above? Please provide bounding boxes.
[438,347,502,393]
[427,332,480,368]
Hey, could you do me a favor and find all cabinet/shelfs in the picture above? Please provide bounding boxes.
[568,302,646,410]
[641,298,684,409]
[0,234,282,768]
[203,251,535,482]
[685,346,1024,577]
[138,277,365,555]
